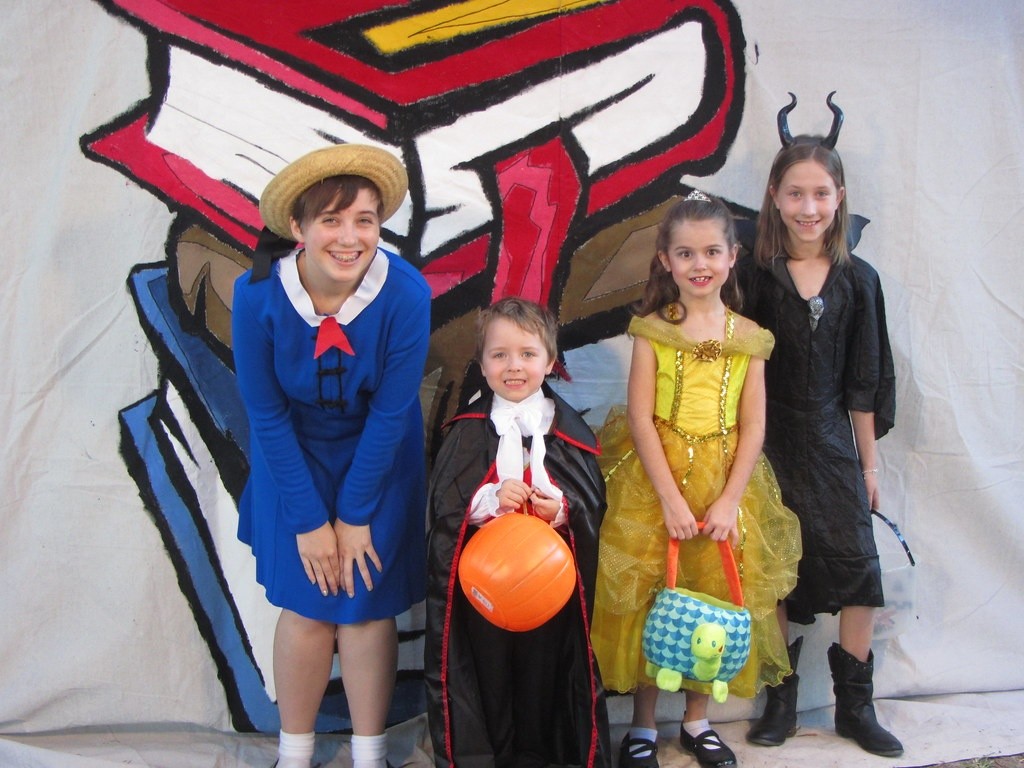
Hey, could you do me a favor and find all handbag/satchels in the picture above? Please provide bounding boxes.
[640,522,754,702]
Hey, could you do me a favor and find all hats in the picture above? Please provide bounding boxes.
[259,143,410,243]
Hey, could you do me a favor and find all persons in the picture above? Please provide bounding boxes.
[722,91,908,758]
[425,298,608,766]
[591,192,802,768]
[232,143,433,768]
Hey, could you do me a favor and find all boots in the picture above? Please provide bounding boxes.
[827,642,906,759]
[746,635,805,746]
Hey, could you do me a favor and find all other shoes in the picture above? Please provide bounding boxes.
[620,731,661,768]
[679,719,738,768]
